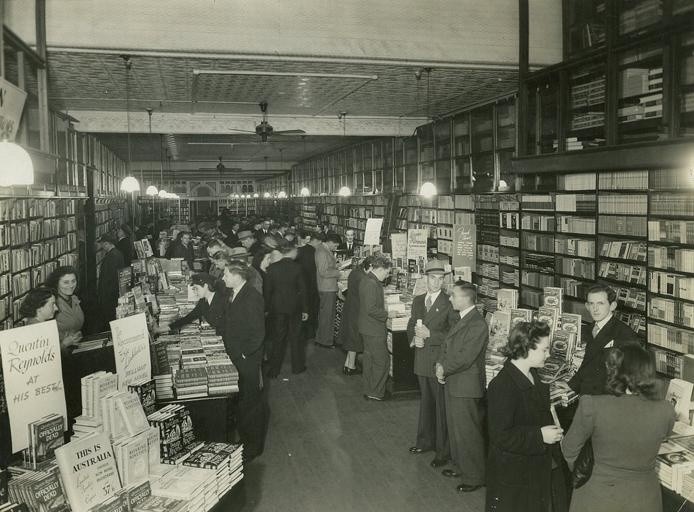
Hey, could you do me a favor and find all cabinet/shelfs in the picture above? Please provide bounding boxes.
[2,201,134,331]
[520,1,694,430]
[230,89,521,400]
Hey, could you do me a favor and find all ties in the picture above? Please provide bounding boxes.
[426,296,432,312]
[349,244,351,251]
[592,324,599,338]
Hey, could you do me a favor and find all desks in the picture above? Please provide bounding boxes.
[658,481,694,511]
[207,477,249,512]
[113,394,244,447]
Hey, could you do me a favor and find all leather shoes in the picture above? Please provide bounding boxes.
[343,366,362,376]
[410,444,485,491]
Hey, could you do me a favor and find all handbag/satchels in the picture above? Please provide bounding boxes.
[572,437,593,489]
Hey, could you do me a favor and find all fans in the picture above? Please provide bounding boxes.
[197,156,243,178]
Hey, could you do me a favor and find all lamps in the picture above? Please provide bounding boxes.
[0,25,181,200]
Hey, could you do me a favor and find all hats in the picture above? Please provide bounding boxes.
[190,274,217,286]
[237,230,255,243]
[422,260,453,275]
[229,247,251,258]
